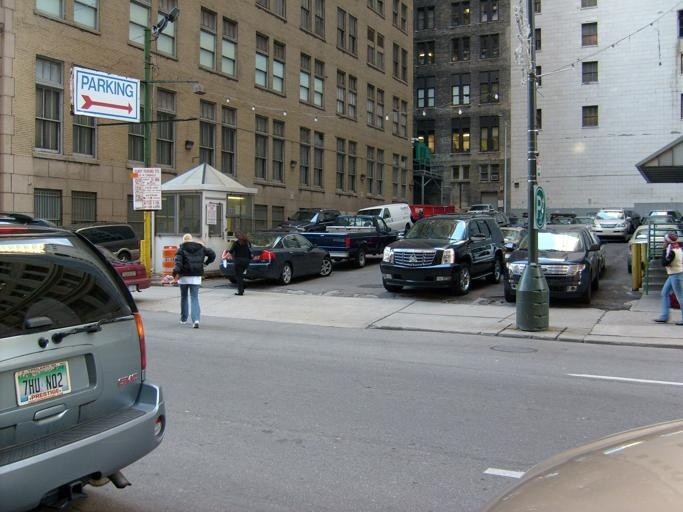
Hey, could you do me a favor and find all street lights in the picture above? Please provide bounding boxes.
[137,75,207,273]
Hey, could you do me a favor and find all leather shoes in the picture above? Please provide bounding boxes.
[675,320,683,326]
[653,317,670,323]
[235,289,245,296]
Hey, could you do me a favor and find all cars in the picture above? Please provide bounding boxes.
[479,418,683,512]
[503,227,600,304]
[92,244,150,292]
[218,231,332,286]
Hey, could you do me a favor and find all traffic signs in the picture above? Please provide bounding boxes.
[71,67,140,125]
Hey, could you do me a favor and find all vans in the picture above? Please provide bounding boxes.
[60,222,140,263]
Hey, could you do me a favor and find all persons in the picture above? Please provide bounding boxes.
[222,230,252,295]
[654,232,683,325]
[172,233,216,328]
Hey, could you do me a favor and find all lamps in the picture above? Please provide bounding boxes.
[359,173,364,181]
[290,160,296,168]
[184,139,193,149]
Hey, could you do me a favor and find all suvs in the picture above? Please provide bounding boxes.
[0,210,165,511]
[379,213,505,296]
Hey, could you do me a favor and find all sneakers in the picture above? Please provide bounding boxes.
[178,320,189,327]
[193,319,200,329]
